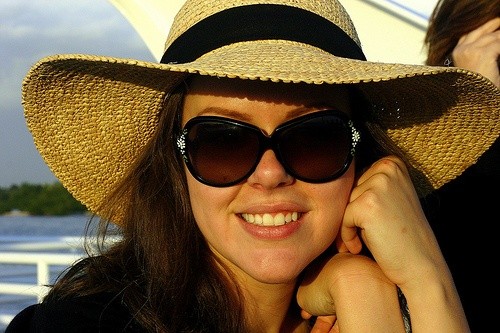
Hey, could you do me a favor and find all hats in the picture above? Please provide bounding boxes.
[17,0,500,231]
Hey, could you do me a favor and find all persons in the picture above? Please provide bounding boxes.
[0,0,472,332]
[417,0,500,333]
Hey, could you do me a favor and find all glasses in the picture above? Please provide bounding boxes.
[169,109,363,186]
